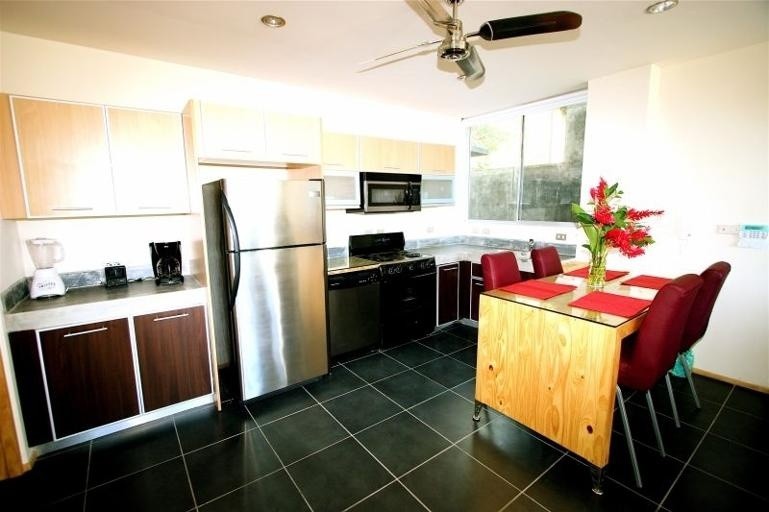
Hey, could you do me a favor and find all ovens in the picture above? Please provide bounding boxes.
[382,261,436,346]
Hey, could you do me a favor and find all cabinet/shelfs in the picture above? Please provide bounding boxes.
[183,98,322,173]
[358,139,419,172]
[105,93,193,215]
[417,143,457,174]
[2,92,114,215]
[322,137,360,174]
[37,288,215,450]
[437,261,484,329]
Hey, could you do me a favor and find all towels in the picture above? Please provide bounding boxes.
[500,280,577,300]
[621,275,672,290]
[564,267,629,282]
[568,290,652,317]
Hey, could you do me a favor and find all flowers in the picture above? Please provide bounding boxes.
[570,177,664,285]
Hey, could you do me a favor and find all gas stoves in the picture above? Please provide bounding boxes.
[357,249,437,282]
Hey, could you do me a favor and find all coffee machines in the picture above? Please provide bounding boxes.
[148,241,184,286]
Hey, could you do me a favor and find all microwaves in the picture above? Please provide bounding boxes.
[345,172,421,215]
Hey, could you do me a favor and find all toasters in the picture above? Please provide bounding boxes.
[104,262,128,289]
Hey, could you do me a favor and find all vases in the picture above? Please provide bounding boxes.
[588,247,608,291]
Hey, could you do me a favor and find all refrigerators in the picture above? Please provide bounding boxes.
[201,177,331,406]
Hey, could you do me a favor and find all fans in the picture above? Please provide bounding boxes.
[358,0,582,81]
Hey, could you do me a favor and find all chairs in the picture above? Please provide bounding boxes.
[531,246,563,278]
[480,252,522,290]
[615,273,702,489]
[666,261,731,428]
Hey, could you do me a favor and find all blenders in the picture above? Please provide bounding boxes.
[24,238,67,299]
